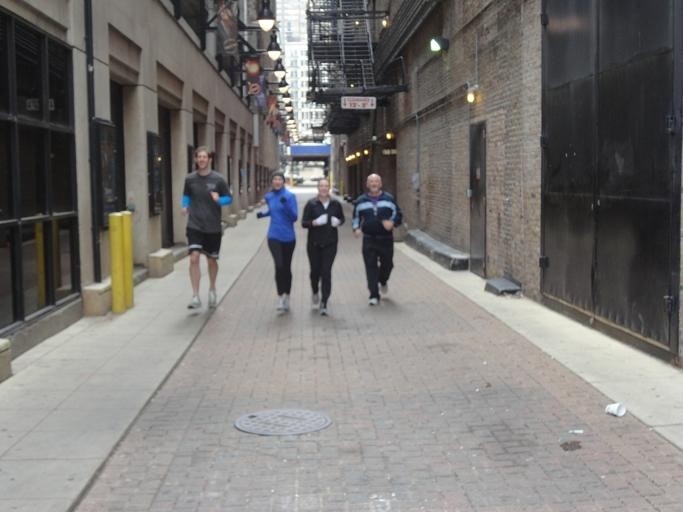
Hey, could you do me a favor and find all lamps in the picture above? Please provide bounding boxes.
[427,34,451,54]
[464,84,479,104]
[205,0,296,125]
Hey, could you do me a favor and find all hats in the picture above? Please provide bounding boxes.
[270,172,285,184]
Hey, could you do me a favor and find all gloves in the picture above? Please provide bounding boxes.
[330,215,341,228]
[313,213,328,226]
[255,211,263,219]
[279,196,287,203]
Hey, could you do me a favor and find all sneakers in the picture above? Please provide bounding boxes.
[368,296,378,305]
[310,289,319,305]
[187,294,201,308]
[319,299,328,316]
[379,284,389,295]
[207,291,217,308]
[275,294,290,313]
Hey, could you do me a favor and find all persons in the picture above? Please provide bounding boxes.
[179,146,233,313]
[256,171,298,312]
[350,174,401,306]
[300,178,346,316]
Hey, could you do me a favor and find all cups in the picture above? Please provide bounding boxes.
[605,401,626,416]
